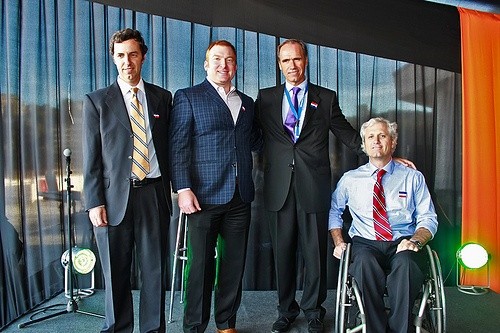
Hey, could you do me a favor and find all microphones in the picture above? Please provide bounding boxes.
[63,148,72,166]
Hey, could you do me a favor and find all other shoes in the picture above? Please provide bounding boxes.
[216,325,236,333]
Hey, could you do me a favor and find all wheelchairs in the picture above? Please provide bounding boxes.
[334,221,447,333]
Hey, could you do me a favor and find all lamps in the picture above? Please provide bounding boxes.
[61,245,96,299]
[456,243,491,296]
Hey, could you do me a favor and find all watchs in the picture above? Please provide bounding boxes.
[410,239,423,250]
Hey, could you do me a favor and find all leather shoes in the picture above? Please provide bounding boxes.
[308,317,323,333]
[271,315,292,333]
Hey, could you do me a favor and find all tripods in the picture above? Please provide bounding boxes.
[17,161,106,329]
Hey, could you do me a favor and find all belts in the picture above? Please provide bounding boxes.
[130,177,162,190]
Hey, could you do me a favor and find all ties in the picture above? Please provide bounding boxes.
[129,87,150,181]
[284,86,300,143]
[373,169,393,241]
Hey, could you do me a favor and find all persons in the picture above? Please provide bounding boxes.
[328,118,439,333]
[81,29,173,333]
[254,39,417,333]
[172,40,263,333]
